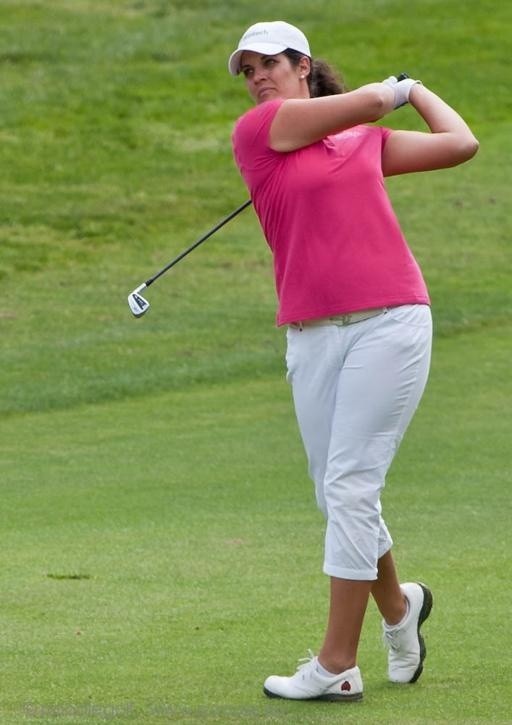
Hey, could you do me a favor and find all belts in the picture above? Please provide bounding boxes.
[292,307,382,325]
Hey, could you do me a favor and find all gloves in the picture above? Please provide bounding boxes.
[383,76,422,110]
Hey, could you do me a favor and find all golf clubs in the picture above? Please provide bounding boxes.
[126,74,408,317]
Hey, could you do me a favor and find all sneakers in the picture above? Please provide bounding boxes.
[263,657,363,702]
[382,582,432,683]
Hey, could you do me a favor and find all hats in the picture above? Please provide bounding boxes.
[228,21,311,75]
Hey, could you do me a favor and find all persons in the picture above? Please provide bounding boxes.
[226,18,478,707]
